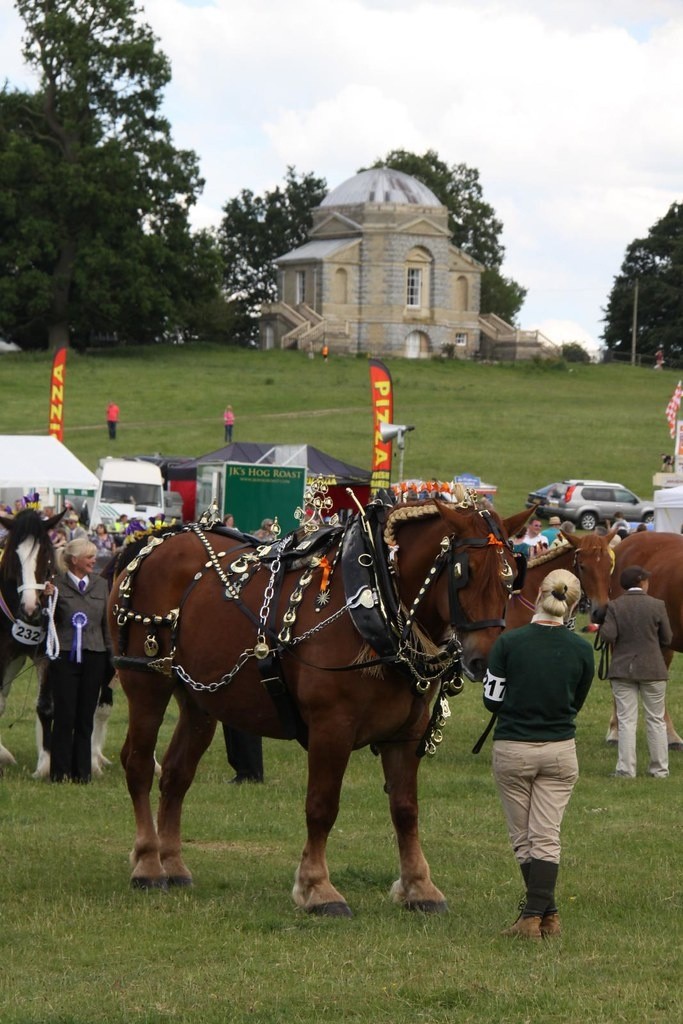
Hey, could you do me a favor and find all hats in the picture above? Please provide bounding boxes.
[560,521,576,537]
[620,565,652,589]
[63,514,78,522]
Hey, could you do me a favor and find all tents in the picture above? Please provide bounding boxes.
[166,442,374,525]
[0,434,101,535]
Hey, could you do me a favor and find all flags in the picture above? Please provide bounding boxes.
[664,380,683,440]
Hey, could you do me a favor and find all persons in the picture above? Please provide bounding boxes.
[222,513,275,543]
[599,565,674,780]
[43,537,114,785]
[322,344,329,362]
[105,399,120,441]
[654,348,664,371]
[660,453,674,472]
[223,404,235,444]
[513,511,648,558]
[0,493,182,561]
[482,568,596,942]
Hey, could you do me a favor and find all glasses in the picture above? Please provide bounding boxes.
[535,524,542,528]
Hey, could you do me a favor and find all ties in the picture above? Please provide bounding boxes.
[79,580,85,593]
[70,532,73,540]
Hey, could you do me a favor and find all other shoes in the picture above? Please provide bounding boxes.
[500,900,542,941]
[540,913,561,938]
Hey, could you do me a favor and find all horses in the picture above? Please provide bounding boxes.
[0,493,683,919]
